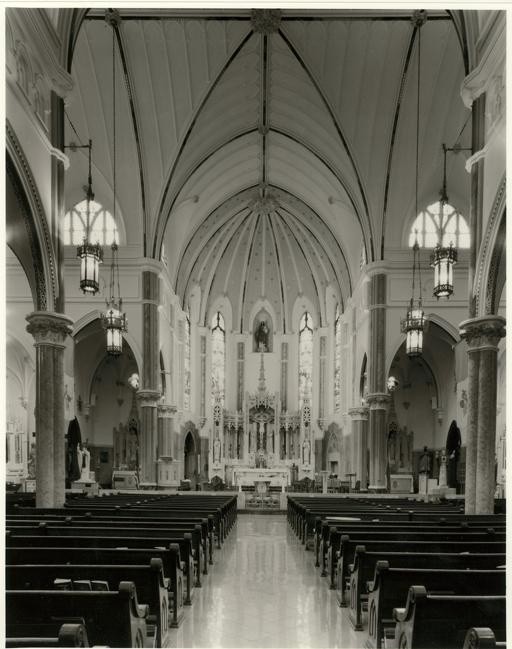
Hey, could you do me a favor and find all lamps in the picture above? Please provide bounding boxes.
[384,375,400,392]
[403,143,457,358]
[65,135,128,356]
[128,373,141,389]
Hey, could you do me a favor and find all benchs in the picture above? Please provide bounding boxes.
[287,495,506,649]
[8,491,239,645]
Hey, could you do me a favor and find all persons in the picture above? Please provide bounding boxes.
[303,438,311,466]
[257,321,268,348]
[252,417,273,437]
[435,445,457,486]
[212,437,220,463]
[76,443,93,481]
